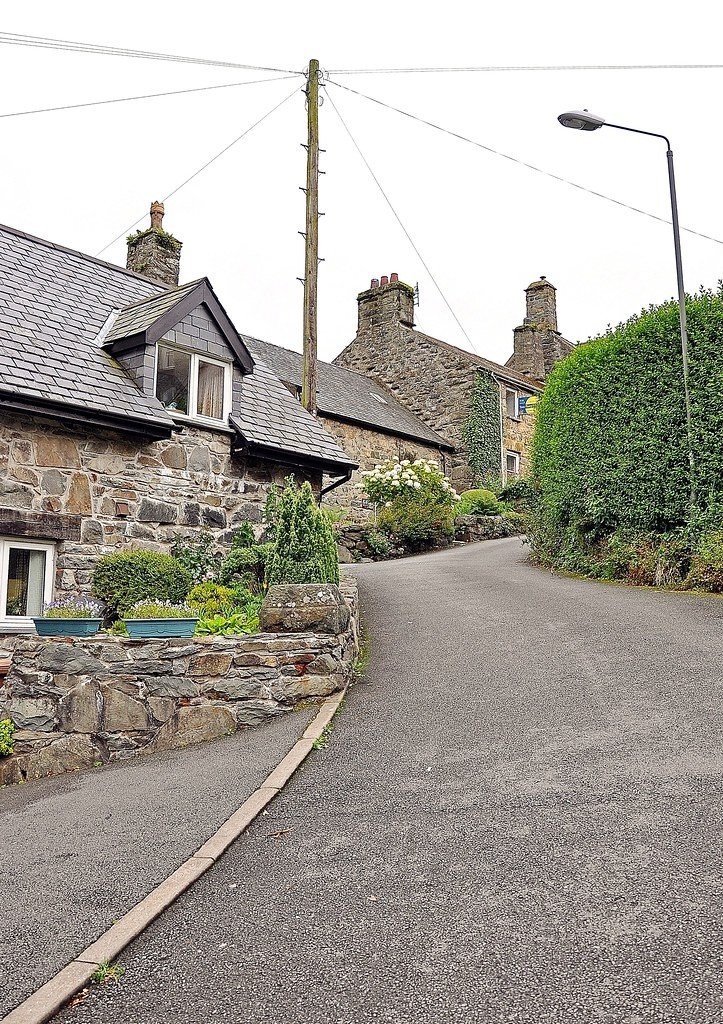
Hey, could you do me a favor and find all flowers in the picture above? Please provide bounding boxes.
[37,593,107,618]
[120,595,199,618]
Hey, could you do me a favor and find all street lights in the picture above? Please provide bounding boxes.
[556,107,697,509]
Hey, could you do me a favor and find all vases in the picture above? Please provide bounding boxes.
[30,618,105,636]
[118,615,201,639]
